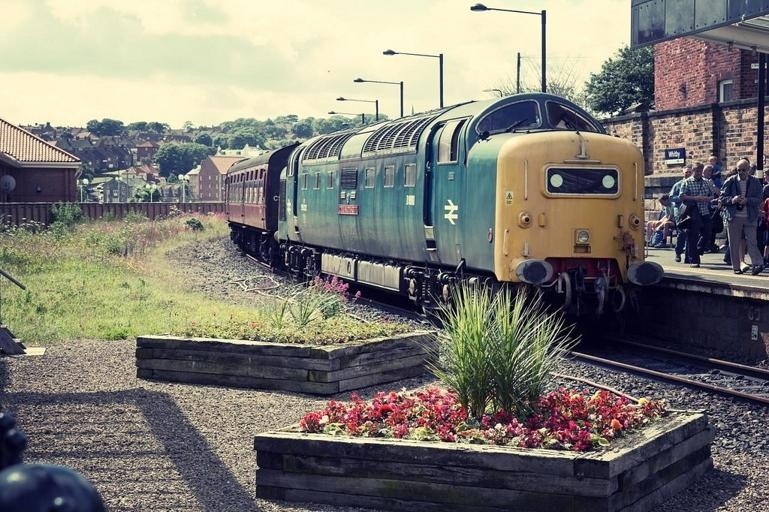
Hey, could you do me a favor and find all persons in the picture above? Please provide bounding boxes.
[643,155,769,276]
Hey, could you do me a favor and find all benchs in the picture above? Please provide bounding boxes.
[661,219,727,248]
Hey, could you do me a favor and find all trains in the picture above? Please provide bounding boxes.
[224,92,667,340]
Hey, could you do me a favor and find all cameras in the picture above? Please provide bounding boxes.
[736,197,744,210]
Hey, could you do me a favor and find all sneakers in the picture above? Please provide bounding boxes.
[723,258,769,277]
[674,244,719,268]
[654,239,667,248]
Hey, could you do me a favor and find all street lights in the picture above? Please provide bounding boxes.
[328,77,404,124]
[469,2,549,95]
[382,49,443,110]
